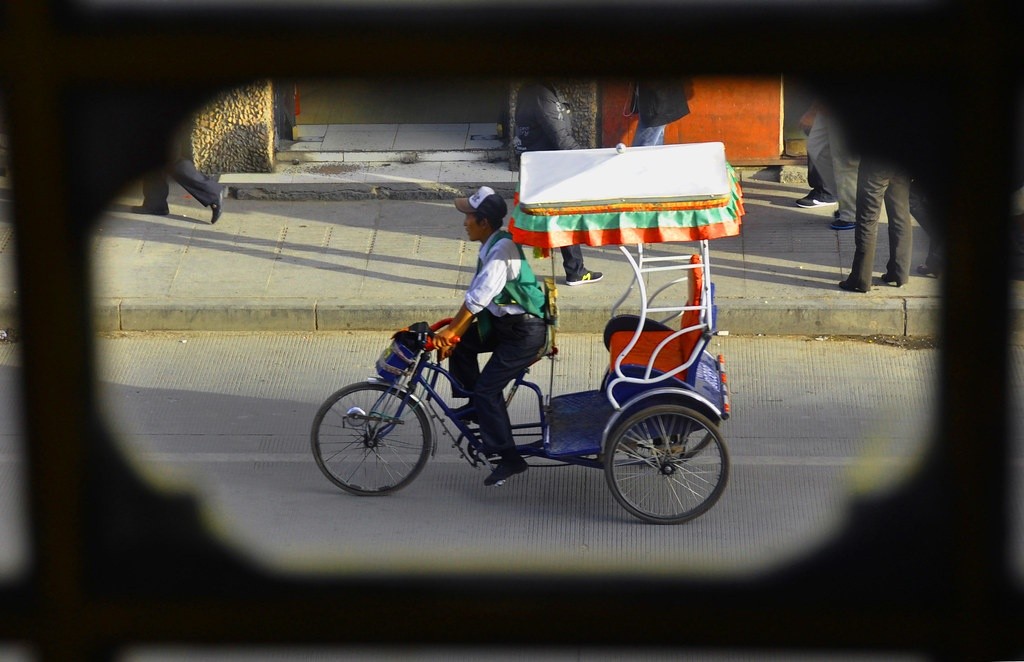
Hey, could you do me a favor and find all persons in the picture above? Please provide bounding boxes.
[840,159,906,292]
[429,185,549,487]
[805,110,862,229]
[529,89,604,285]
[131,156,224,222]
[794,101,837,206]
[627,85,693,147]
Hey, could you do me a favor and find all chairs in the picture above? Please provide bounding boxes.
[604,254,705,397]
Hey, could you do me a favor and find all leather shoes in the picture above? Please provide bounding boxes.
[210,183,228,225]
[130,202,170,214]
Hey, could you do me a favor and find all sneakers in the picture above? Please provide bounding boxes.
[484,458,528,486]
[446,402,482,426]
[829,219,857,231]
[834,210,840,219]
[796,192,837,209]
[566,269,603,286]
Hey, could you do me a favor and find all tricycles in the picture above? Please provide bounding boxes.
[306,142,747,531]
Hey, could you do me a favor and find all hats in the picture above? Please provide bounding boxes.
[454,185,508,218]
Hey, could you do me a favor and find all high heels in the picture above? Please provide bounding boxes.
[917,265,940,279]
[881,273,905,288]
[839,279,868,294]
